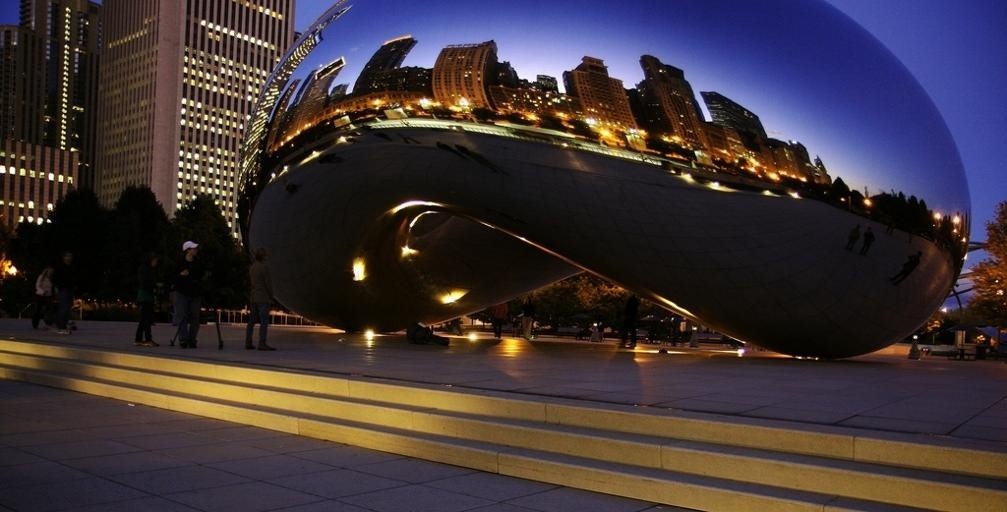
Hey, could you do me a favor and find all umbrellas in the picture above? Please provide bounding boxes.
[947,322,971,344]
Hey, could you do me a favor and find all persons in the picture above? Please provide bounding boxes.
[973,339,1006,360]
[446,292,739,350]
[843,215,962,286]
[31,239,277,352]
[279,129,509,197]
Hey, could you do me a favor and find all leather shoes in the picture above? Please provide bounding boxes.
[259,344,276,351]
[245,344,256,350]
[145,340,160,347]
[134,341,148,346]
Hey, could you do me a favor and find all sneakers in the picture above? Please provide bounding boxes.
[180,341,189,348]
[189,343,197,348]
[58,328,72,337]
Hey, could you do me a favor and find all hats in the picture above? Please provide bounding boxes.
[183,241,199,251]
